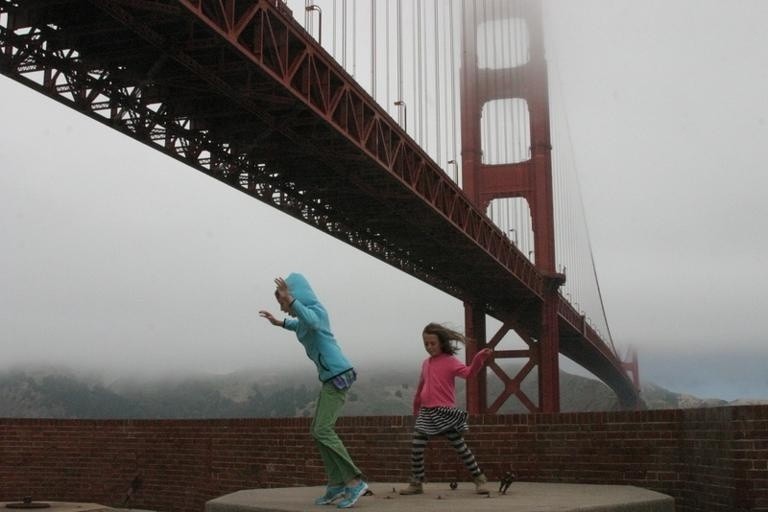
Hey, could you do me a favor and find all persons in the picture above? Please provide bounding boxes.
[256,272,370,510]
[397,320,497,495]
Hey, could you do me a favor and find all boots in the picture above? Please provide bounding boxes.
[399,479,423,495]
[476,473,490,494]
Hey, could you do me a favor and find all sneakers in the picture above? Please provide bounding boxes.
[336,479,369,509]
[315,486,345,505]
